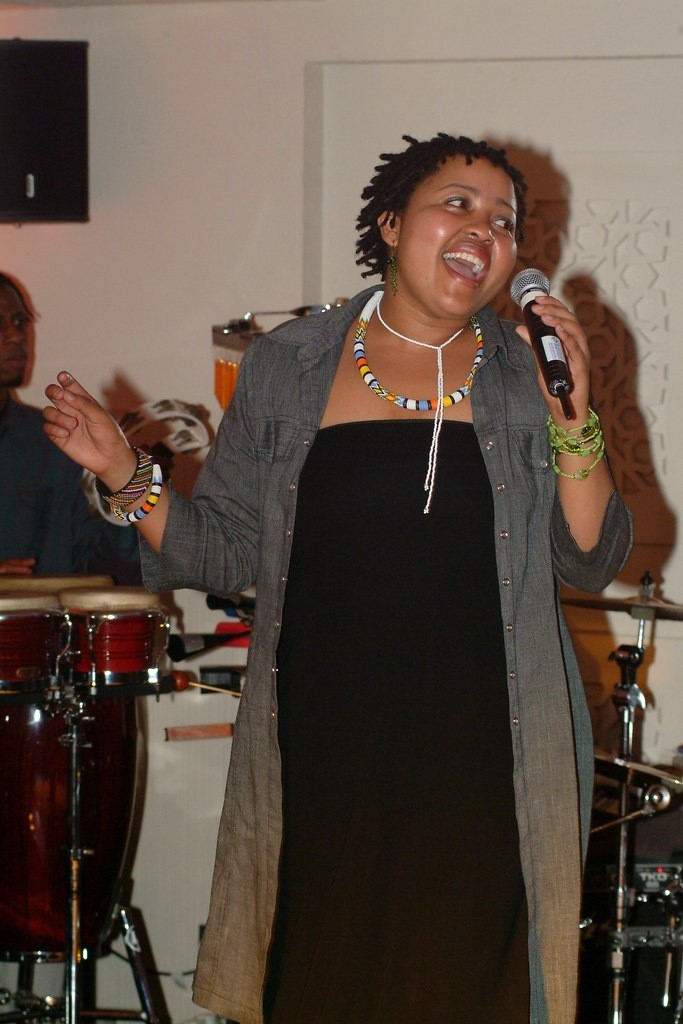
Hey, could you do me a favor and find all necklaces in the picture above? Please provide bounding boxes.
[353,290,483,411]
[376,297,464,515]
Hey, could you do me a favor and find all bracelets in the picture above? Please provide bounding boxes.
[544,407,606,480]
[109,456,161,522]
[96,447,151,508]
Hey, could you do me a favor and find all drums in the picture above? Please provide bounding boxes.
[58,585,161,688]
[0,590,69,696]
[0,573,139,965]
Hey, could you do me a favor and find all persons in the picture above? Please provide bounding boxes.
[44,134,632,1024]
[0,272,140,586]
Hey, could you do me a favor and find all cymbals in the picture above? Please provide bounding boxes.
[79,399,214,528]
[558,594,683,622]
